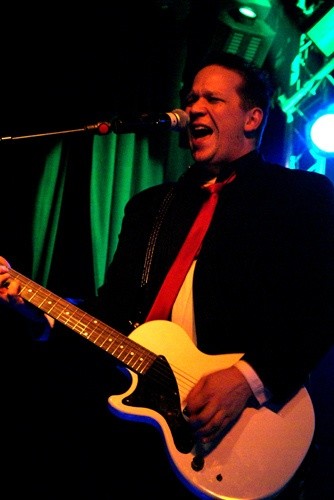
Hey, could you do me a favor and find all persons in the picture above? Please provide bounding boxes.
[0,48,334,500]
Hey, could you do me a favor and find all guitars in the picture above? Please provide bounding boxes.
[0,264,316,500]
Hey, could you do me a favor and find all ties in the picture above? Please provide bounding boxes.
[136,173,237,330]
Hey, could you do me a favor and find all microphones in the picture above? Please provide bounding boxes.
[110,108,191,134]
